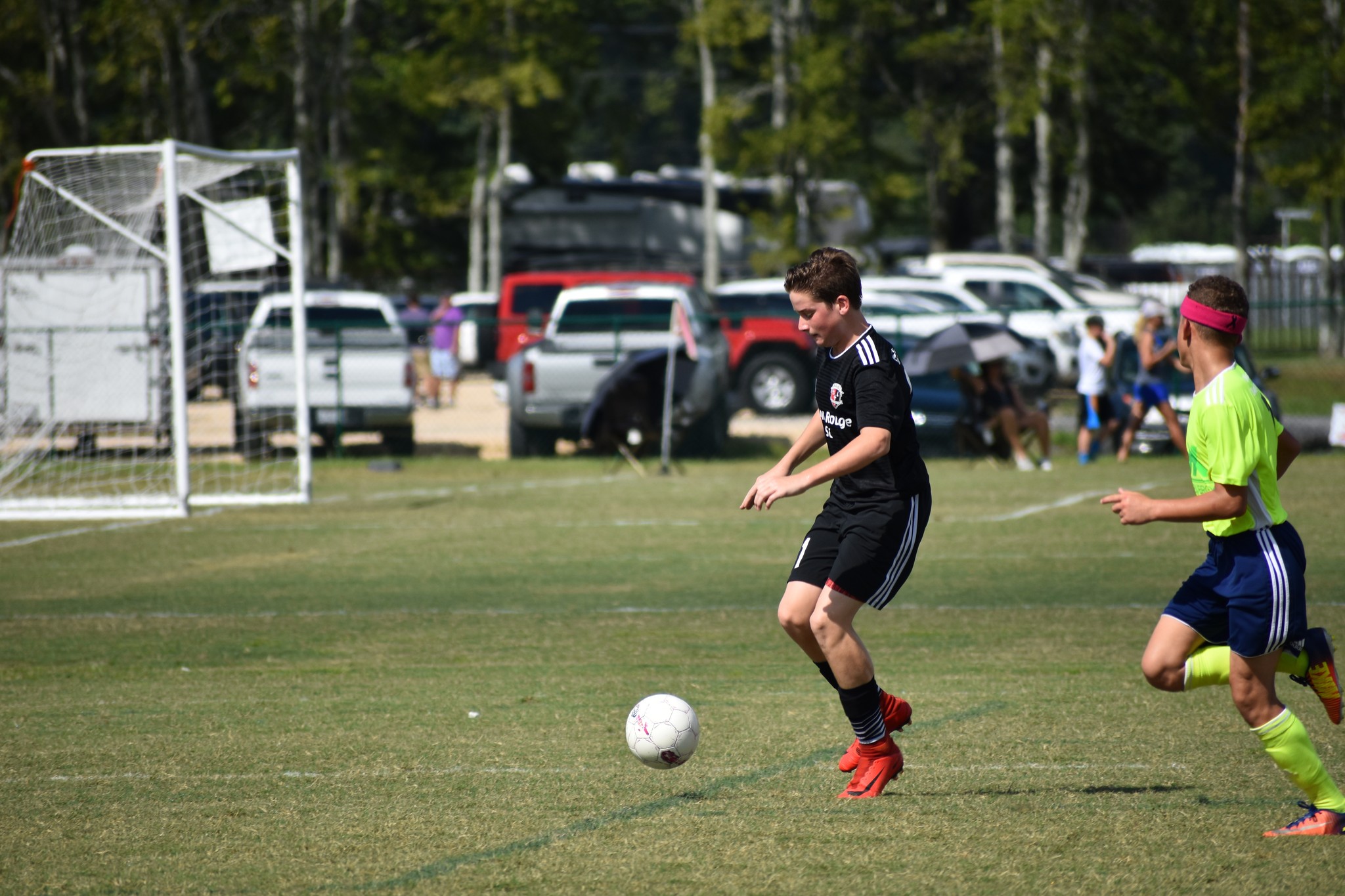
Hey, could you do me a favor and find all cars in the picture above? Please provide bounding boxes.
[167,277,281,402]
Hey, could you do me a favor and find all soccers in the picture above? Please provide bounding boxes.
[625,694,700,770]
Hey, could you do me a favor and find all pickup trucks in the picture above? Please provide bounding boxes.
[228,288,419,461]
[385,172,1345,463]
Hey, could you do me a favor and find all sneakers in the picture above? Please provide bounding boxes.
[837,732,904,800]
[1290,627,1345,724]
[839,691,912,773]
[1261,801,1345,838]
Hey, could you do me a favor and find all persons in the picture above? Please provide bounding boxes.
[1099,275,1345,836]
[388,281,467,409]
[738,245,932,802]
[980,297,1193,470]
